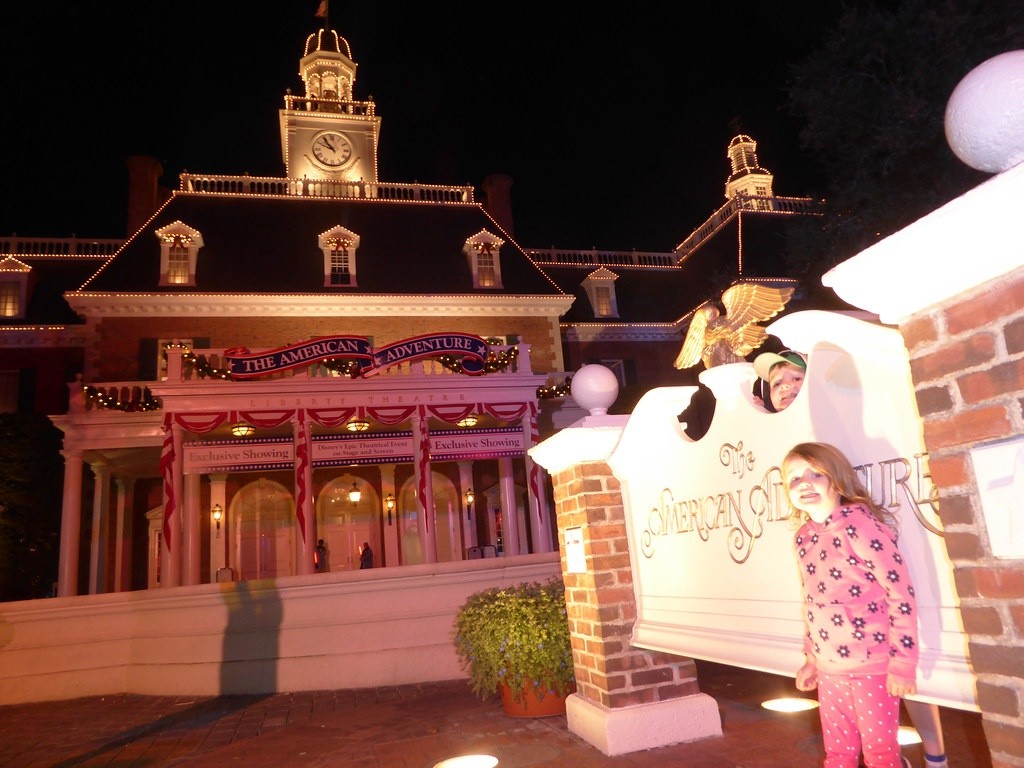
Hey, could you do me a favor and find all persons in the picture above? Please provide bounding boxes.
[781,442,919,768]
[360,541,374,569]
[316,540,326,568]
[752,349,948,768]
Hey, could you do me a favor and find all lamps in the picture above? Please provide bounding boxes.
[347,420,370,431]
[211,503,222,538]
[349,481,361,509]
[466,488,474,520]
[385,493,395,525]
[232,425,256,437]
[456,414,479,427]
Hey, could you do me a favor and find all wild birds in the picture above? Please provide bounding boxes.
[672,281,798,370]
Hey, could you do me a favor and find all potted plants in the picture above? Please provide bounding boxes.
[449,575,576,718]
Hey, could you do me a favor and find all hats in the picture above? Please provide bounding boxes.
[752,352,806,382]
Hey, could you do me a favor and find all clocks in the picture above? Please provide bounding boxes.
[310,130,356,171]
